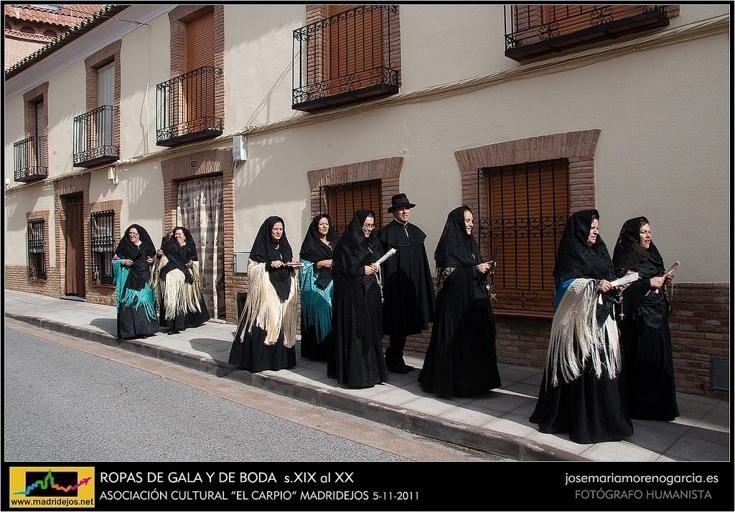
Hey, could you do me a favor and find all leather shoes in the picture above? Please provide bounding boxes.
[390,363,409,373]
[405,365,414,371]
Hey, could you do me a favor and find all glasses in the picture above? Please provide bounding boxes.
[363,224,376,231]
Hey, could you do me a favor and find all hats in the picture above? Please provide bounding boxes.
[388,193,416,214]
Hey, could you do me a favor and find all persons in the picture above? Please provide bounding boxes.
[379,193,437,374]
[325,211,393,389]
[230,216,302,370]
[528,209,639,444]
[157,226,201,335]
[614,216,680,423]
[416,204,505,399]
[110,224,161,341]
[292,214,336,361]
